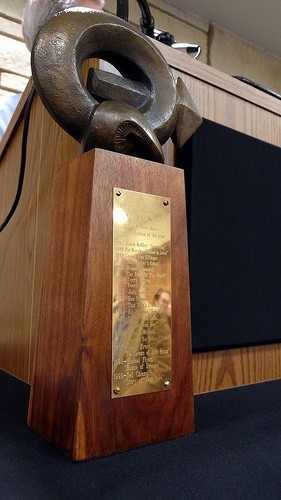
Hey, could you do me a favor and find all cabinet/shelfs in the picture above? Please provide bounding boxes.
[1,35,280,463]
